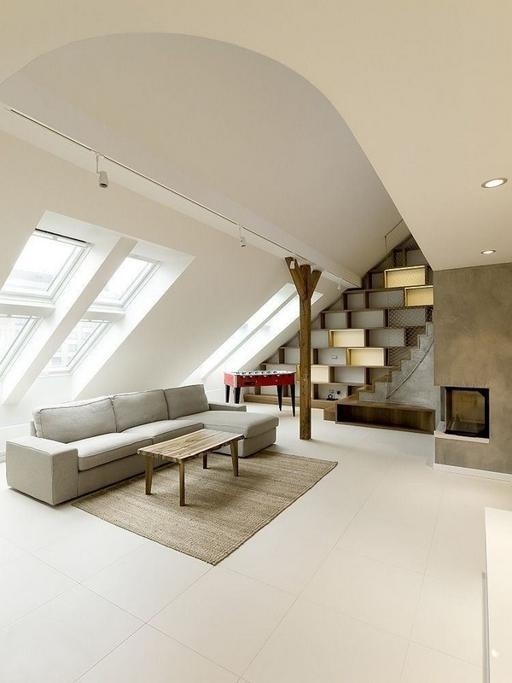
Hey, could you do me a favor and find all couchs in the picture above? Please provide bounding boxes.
[5,401,279,506]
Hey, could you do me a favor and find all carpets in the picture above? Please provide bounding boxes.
[71,449,339,566]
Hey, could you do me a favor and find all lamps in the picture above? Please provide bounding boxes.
[9,108,361,292]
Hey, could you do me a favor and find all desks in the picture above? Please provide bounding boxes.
[224,370,298,417]
[478,503,512,683]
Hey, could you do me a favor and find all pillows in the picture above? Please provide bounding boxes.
[33,383,210,443]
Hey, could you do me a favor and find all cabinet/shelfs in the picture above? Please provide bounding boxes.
[243,235,436,435]
